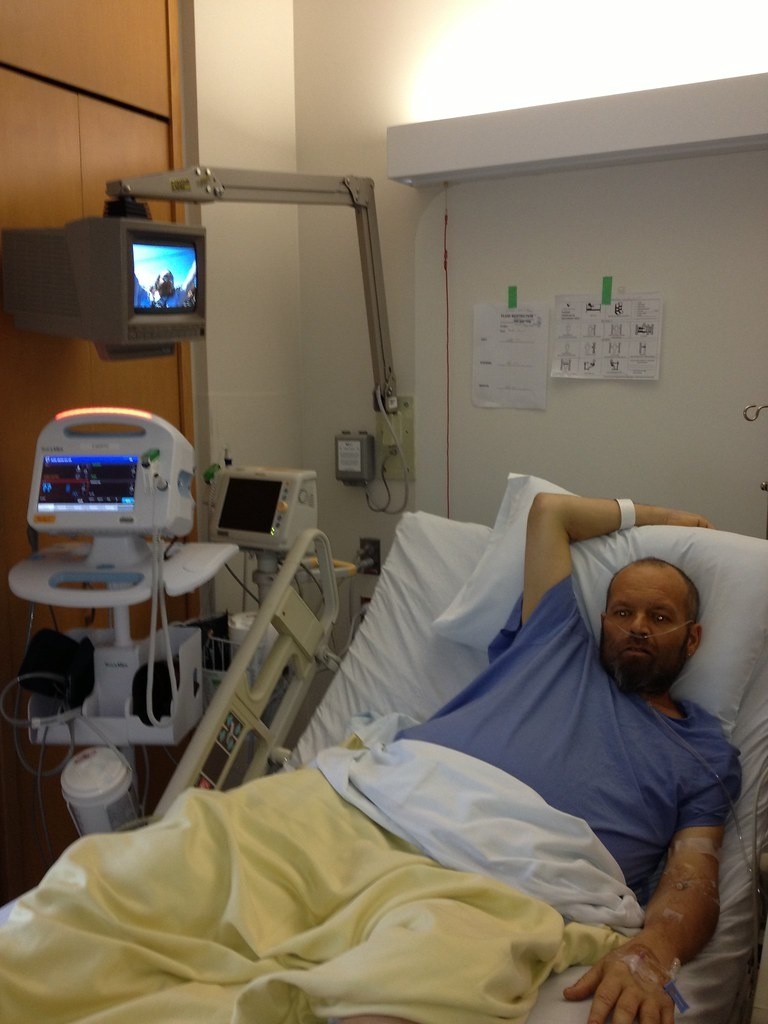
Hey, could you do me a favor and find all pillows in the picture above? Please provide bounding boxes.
[428,465,768,739]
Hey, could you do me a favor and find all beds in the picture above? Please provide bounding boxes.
[148,503,768,1024]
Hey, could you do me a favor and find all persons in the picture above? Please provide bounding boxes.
[148,269,194,308]
[337,492,743,1024]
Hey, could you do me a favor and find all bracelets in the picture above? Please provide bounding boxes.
[614,498,636,531]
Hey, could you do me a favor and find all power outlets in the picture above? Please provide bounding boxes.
[359,596,372,624]
[360,537,382,576]
[374,396,417,486]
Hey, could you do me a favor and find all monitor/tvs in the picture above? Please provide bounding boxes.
[219,476,283,534]
[38,453,137,512]
[65,215,207,345]
